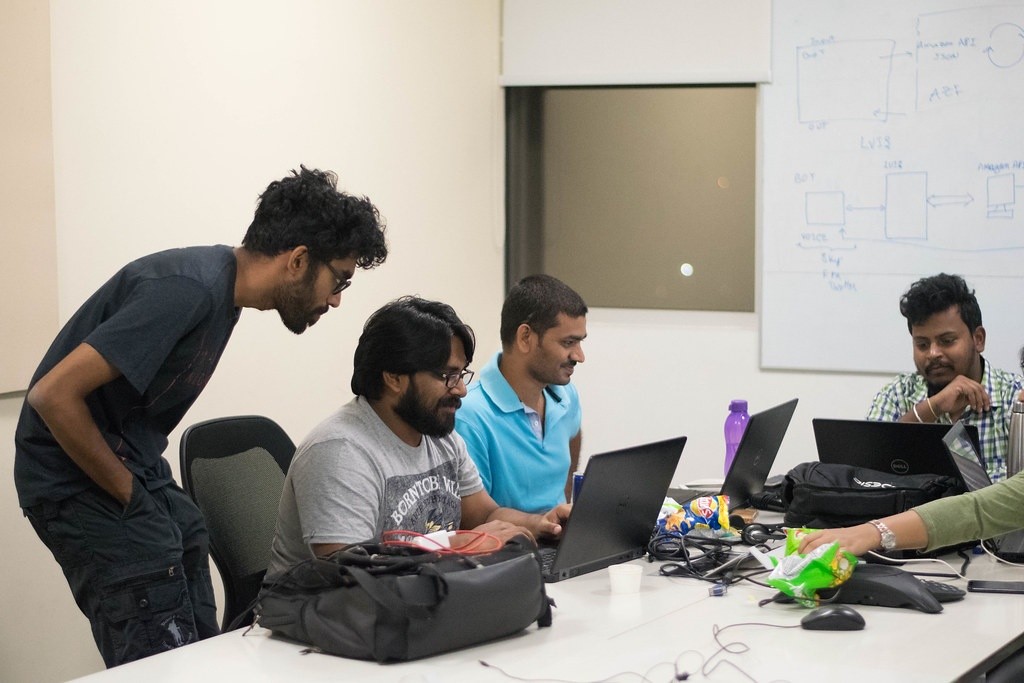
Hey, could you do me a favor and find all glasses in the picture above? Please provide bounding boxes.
[424,366,475,389]
[288,245,351,295]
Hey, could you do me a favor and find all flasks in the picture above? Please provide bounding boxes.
[1007,400,1024,479]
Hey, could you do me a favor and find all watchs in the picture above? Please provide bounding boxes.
[868,519,896,553]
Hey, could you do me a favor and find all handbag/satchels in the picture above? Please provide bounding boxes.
[780,461,965,528]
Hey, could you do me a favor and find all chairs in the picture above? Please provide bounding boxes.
[180,415,300,633]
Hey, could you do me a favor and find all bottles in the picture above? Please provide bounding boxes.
[724,400,751,480]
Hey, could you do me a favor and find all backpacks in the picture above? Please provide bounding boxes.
[242,530,560,666]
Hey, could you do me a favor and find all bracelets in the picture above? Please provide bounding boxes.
[926,397,938,420]
[913,403,923,422]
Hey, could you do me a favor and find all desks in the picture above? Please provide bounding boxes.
[62,520,1024,683]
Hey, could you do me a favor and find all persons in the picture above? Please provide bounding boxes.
[256,295,573,597]
[867,273,1024,483]
[14,166,387,670]
[453,274,589,514]
[798,346,1024,556]
